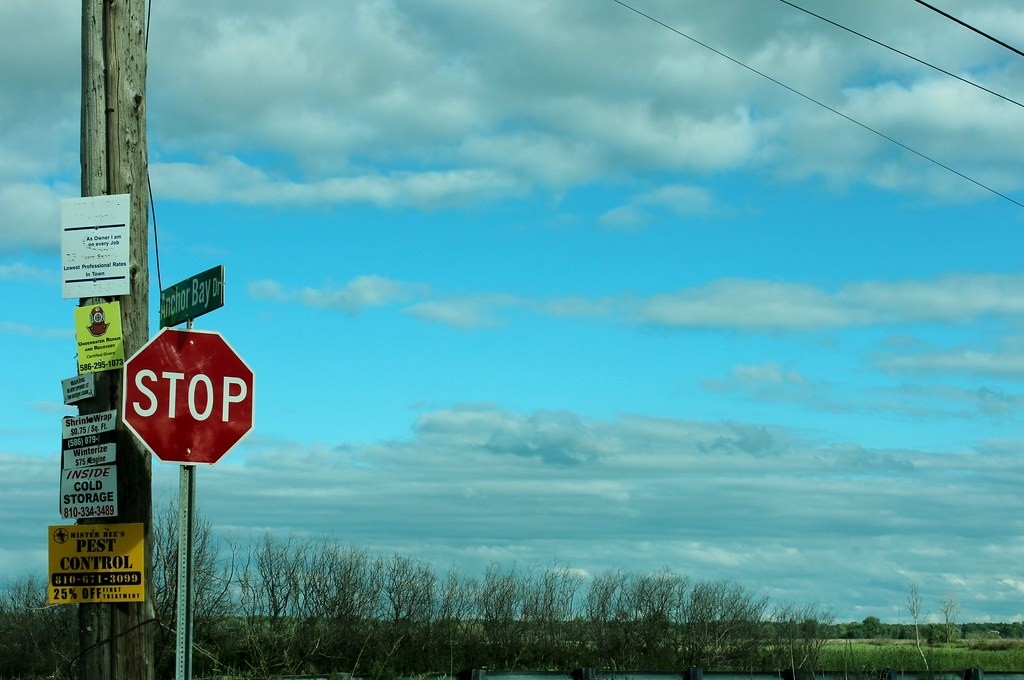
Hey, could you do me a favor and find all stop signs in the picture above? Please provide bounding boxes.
[122,326,254,465]
